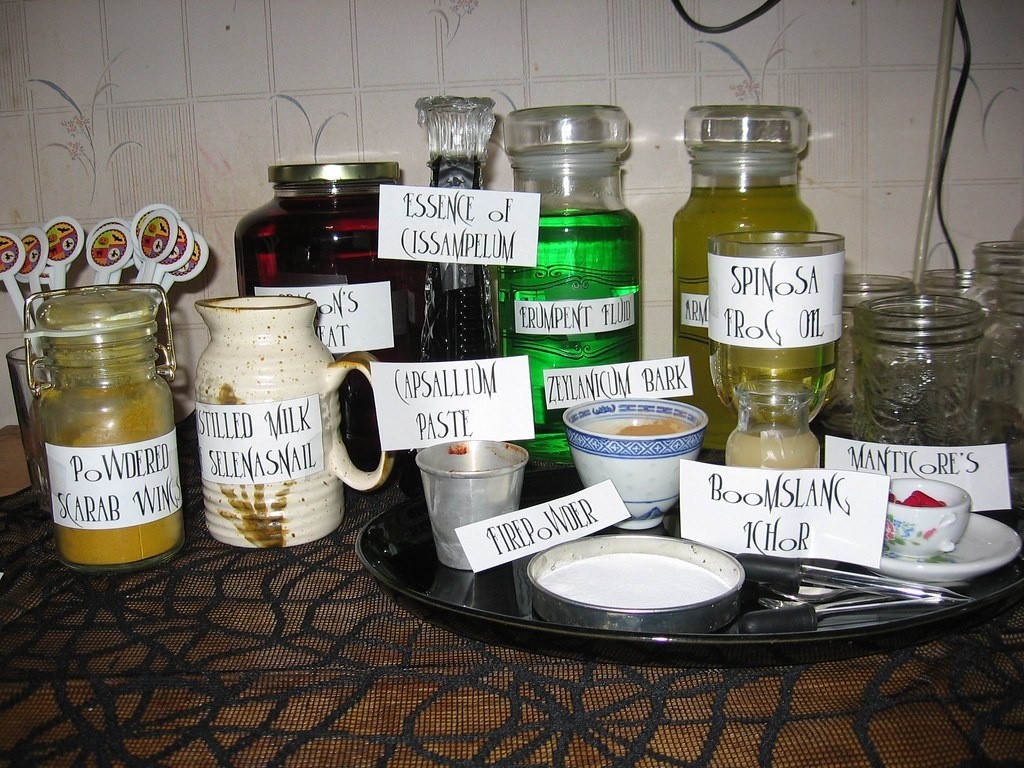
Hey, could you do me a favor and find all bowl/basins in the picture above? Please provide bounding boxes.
[883,478,972,560]
[563,399,708,530]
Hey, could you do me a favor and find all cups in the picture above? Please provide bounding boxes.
[819,239,1024,473]
[7,347,51,496]
[194,296,394,547]
[707,230,845,424]
[416,440,530,572]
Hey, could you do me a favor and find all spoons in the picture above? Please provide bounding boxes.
[758,596,947,619]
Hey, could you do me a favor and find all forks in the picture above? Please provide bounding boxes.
[768,586,923,603]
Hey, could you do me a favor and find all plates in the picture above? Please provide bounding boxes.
[864,513,1022,586]
[526,535,744,635]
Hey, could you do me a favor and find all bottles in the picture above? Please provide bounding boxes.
[498,105,642,464]
[234,161,415,447]
[416,94,498,362]
[725,380,819,468]
[672,106,837,451]
[23,282,187,575]
